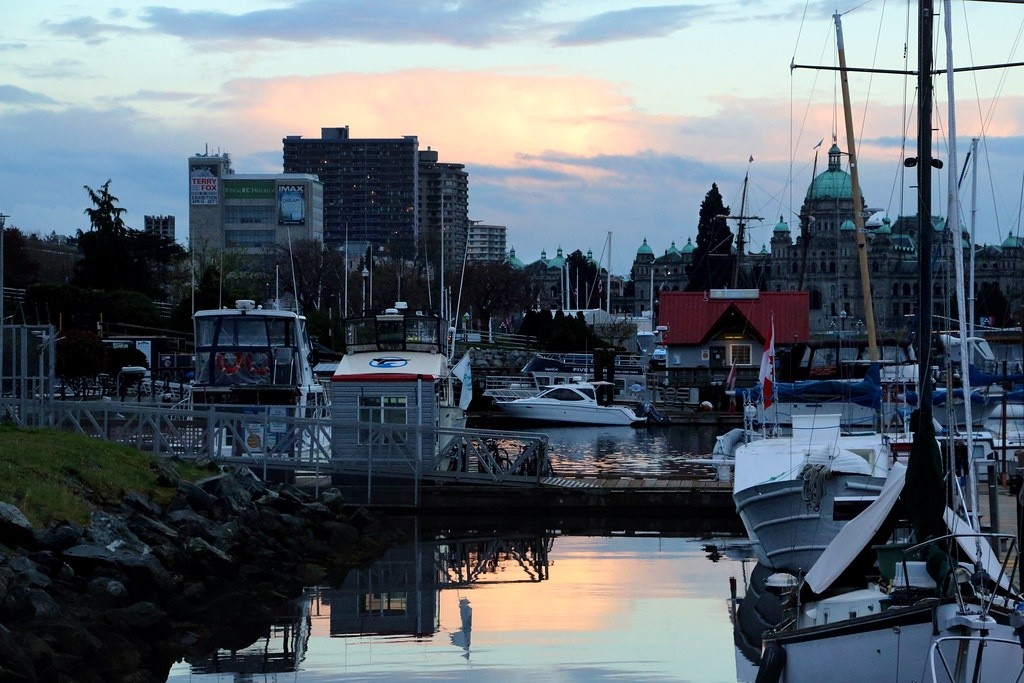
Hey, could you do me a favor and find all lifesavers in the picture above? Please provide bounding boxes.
[217,351,244,374]
[246,350,272,376]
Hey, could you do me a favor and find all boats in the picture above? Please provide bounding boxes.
[482,338,668,428]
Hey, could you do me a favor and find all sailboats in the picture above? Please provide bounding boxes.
[167,2,1024,682]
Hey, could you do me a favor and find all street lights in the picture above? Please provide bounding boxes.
[361,265,369,309]
[839,309,847,331]
